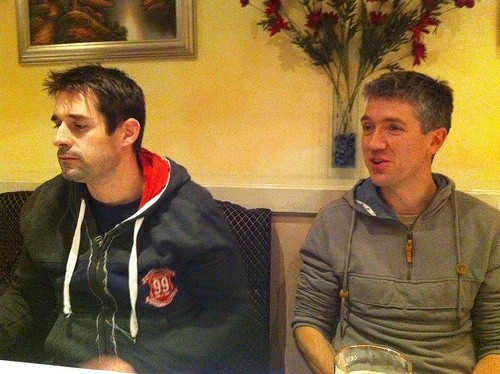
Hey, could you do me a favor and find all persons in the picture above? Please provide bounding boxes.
[290,70,500,374]
[0,61,259,374]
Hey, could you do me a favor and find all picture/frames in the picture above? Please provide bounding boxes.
[15,0,198,64]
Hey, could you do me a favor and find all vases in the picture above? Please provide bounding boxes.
[332,80,357,170]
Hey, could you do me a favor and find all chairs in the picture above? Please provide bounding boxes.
[1,189,273,374]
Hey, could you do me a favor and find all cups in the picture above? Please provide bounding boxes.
[334,345,413,374]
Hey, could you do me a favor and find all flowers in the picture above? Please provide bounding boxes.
[236,0,479,134]
